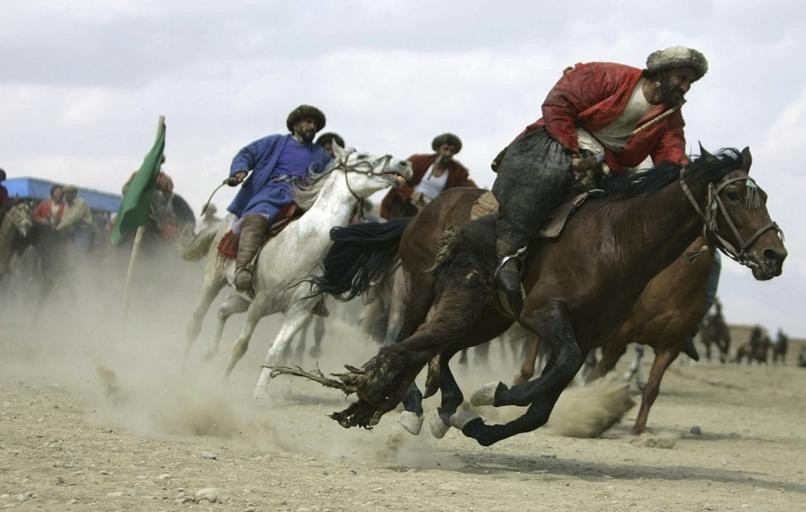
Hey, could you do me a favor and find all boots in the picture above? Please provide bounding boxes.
[234,213,270,288]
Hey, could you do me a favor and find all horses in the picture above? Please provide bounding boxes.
[277,139,787,448]
[177,138,415,401]
[0,194,806,375]
[513,238,722,434]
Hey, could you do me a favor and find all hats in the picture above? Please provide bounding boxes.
[287,105,325,132]
[316,133,344,147]
[432,133,461,154]
[646,46,708,80]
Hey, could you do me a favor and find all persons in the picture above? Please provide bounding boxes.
[0,168,222,280]
[705,301,788,364]
[227,105,336,317]
[366,132,480,286]
[496,45,707,292]
[316,133,345,159]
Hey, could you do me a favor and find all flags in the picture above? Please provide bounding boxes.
[112,123,167,247]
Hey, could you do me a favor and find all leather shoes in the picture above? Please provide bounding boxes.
[497,257,520,295]
